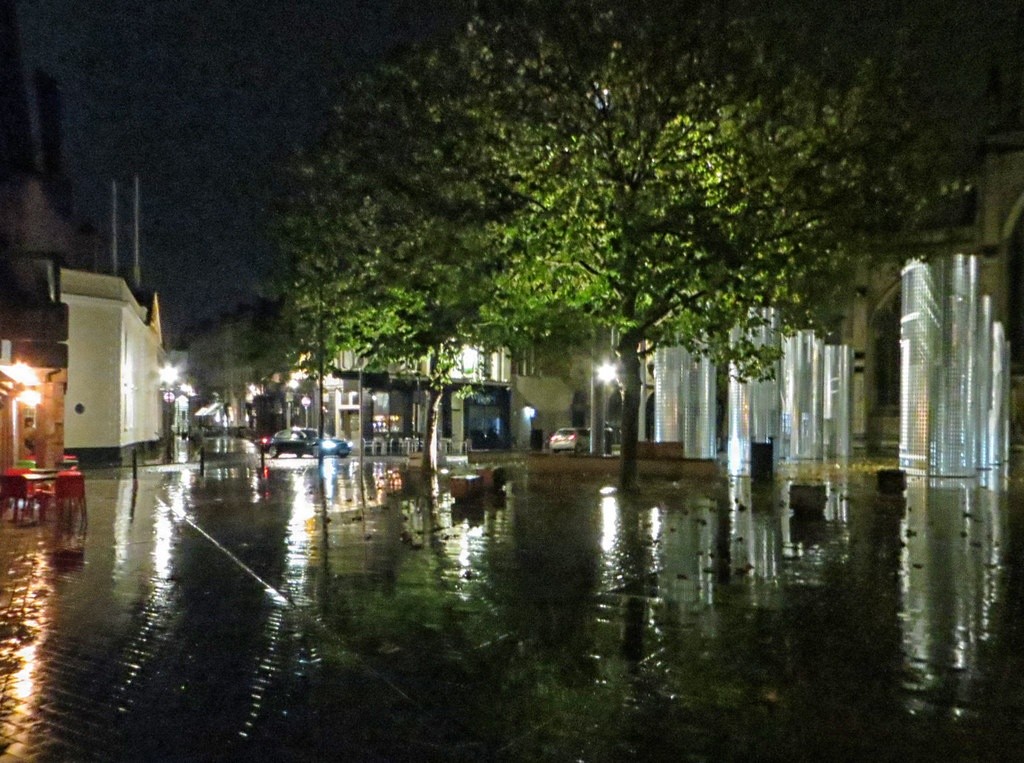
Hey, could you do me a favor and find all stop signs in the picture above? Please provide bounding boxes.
[301,397,311,407]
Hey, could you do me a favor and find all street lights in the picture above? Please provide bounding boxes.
[159,365,177,462]
[598,363,617,455]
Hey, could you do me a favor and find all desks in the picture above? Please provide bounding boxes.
[25,454,76,460]
[32,460,79,469]
[366,440,387,456]
[446,442,468,454]
[401,440,423,454]
[30,469,69,473]
[13,473,72,524]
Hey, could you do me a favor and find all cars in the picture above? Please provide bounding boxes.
[549,428,590,455]
[260,427,352,459]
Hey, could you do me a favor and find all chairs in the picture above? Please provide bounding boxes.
[0,466,88,527]
[361,434,473,457]
